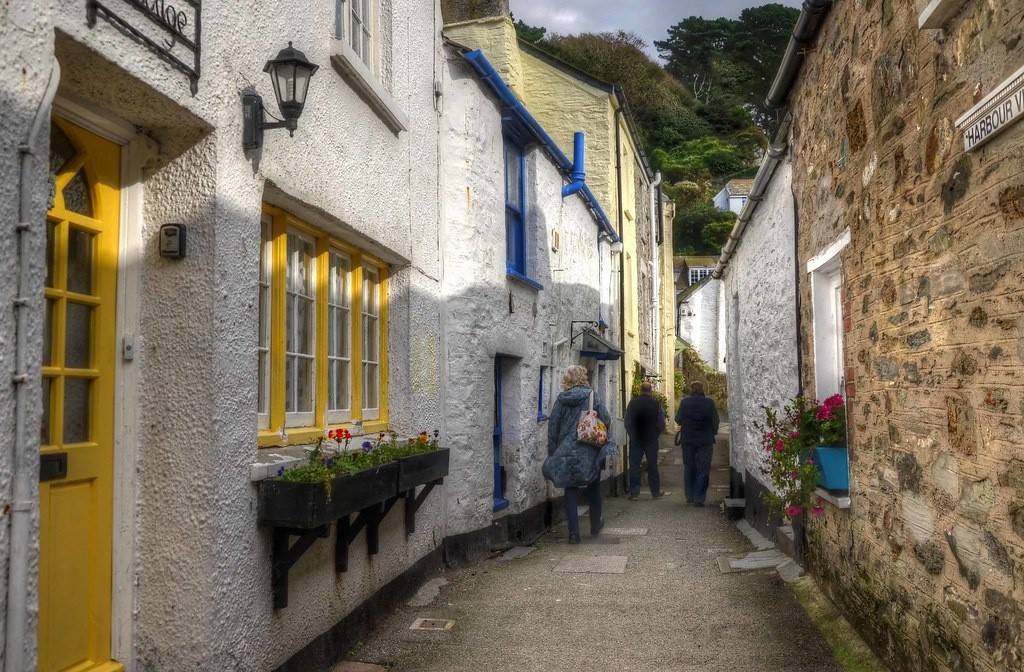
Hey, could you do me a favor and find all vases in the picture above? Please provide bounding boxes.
[259,447,449,528]
[798,447,848,489]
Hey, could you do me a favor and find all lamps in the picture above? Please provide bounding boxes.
[242,41,319,148]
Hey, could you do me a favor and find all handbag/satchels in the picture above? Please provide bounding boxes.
[576,391,607,448]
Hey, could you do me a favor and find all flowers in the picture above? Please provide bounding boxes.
[276,429,440,504]
[754,394,846,522]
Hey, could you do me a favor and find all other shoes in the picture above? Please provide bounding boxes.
[658,490,665,496]
[695,503,705,507]
[591,517,604,535]
[628,495,637,500]
[569,534,580,543]
[687,497,694,503]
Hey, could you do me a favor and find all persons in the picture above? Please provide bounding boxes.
[624,382,666,501]
[675,381,720,507]
[541,365,616,544]
